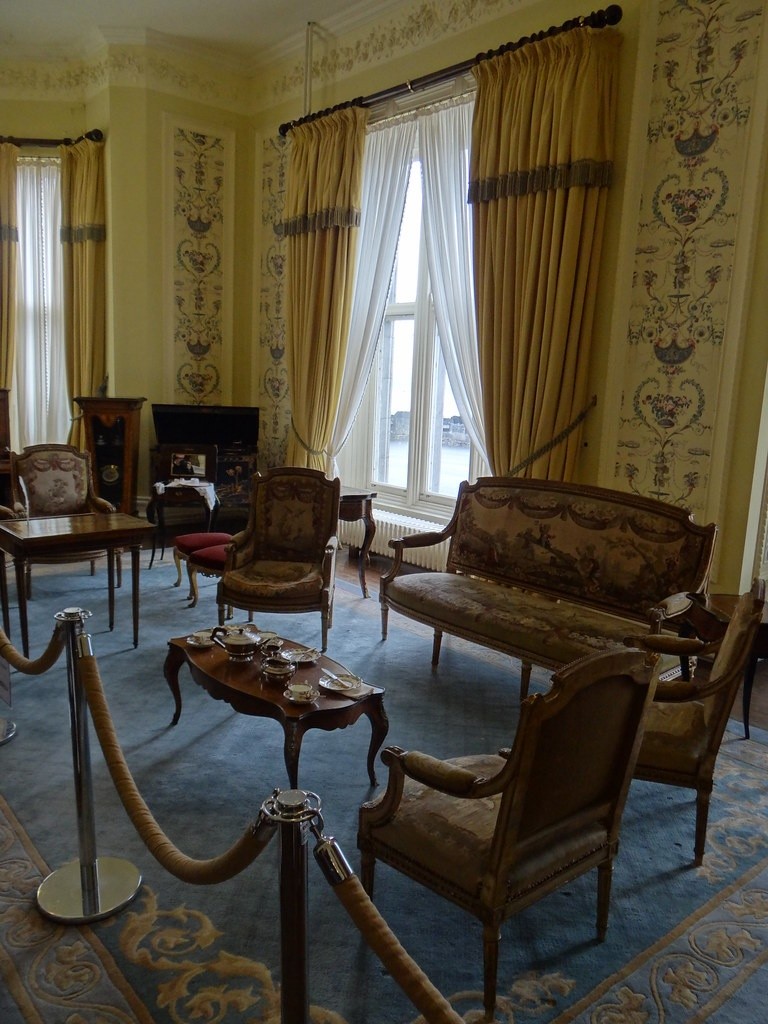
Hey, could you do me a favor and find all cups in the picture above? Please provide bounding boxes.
[187,462,191,469]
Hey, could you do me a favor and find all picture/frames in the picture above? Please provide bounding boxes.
[164,445,218,484]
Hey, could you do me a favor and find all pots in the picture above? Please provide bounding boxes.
[211,628,270,653]
[261,658,297,685]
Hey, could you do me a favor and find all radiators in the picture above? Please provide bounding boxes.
[337,506,450,574]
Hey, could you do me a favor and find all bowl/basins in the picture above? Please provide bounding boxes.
[288,683,312,698]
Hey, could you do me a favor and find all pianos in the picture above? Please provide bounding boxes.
[147,401,260,499]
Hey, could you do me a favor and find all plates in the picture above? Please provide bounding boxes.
[192,631,212,637]
[318,674,361,690]
[257,631,277,639]
[283,688,320,703]
[212,625,255,633]
[281,648,320,661]
[188,637,214,647]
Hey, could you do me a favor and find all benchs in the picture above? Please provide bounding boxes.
[380,475,718,703]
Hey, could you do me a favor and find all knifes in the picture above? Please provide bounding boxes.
[296,648,315,661]
[321,667,349,688]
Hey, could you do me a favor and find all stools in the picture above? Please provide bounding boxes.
[173,533,232,599]
[186,544,232,619]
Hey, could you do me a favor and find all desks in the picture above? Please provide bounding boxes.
[73,397,147,514]
[340,485,378,600]
[0,512,155,660]
[147,482,220,570]
[677,590,768,739]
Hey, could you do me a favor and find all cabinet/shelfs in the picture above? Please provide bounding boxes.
[147,403,262,509]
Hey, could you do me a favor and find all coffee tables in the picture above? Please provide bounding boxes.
[162,622,390,790]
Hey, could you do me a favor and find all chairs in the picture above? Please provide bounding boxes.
[215,464,340,651]
[9,443,123,599]
[514,577,767,867]
[355,647,665,1023]
